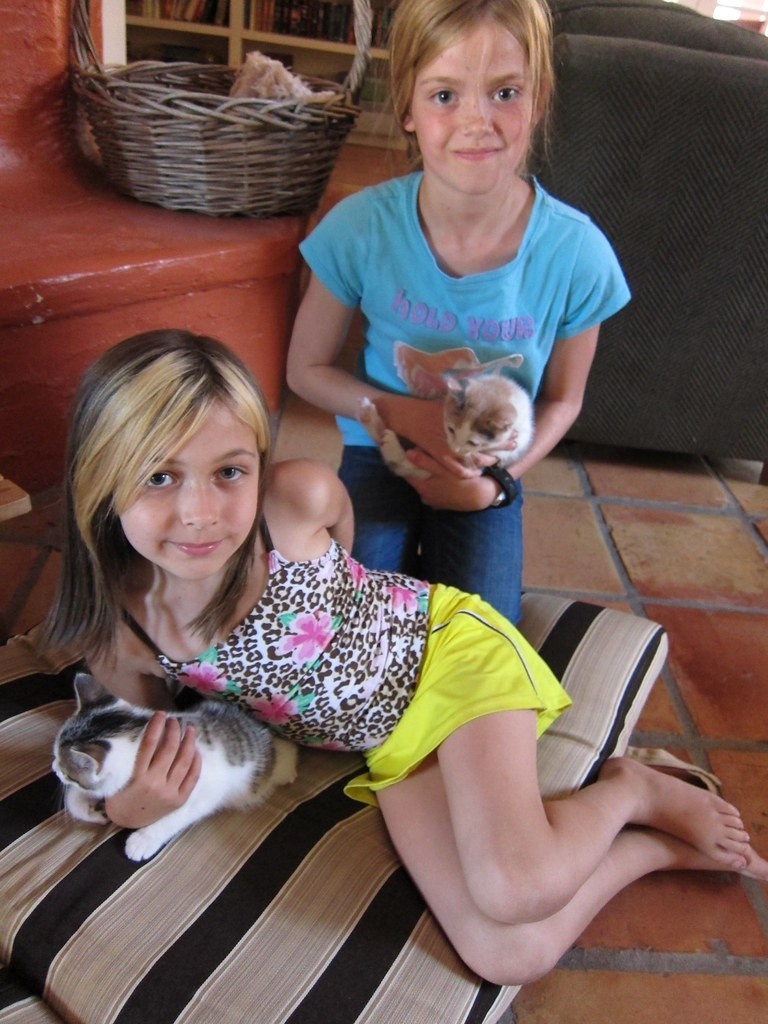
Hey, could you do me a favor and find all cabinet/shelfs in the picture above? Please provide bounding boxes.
[88,0,421,152]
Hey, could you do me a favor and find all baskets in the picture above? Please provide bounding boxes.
[70,0,373,218]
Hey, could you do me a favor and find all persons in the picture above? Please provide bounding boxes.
[41,330,768,987]
[286,0,631,624]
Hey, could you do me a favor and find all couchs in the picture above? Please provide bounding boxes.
[523,0,768,489]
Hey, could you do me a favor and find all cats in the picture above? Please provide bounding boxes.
[357,370,534,479]
[52,673,300,861]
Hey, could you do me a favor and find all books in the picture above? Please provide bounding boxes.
[137,0,398,102]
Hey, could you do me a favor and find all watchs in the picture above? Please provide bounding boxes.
[482,466,519,508]
